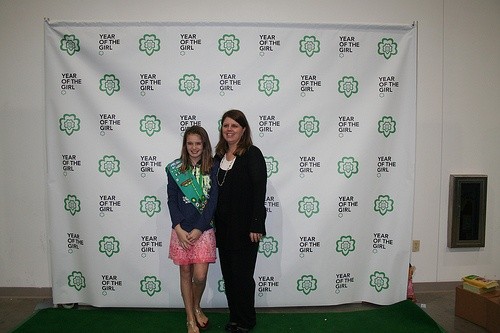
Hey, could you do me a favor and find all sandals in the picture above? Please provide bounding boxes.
[185,306,210,333]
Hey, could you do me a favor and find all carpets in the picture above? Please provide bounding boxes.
[5,297,450,333]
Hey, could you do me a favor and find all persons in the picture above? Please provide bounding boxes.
[211,110,267,333]
[165,126,220,333]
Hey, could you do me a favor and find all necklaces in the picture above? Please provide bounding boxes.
[216,152,235,187]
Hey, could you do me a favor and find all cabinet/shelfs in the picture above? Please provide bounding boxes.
[454,287,500,333]
